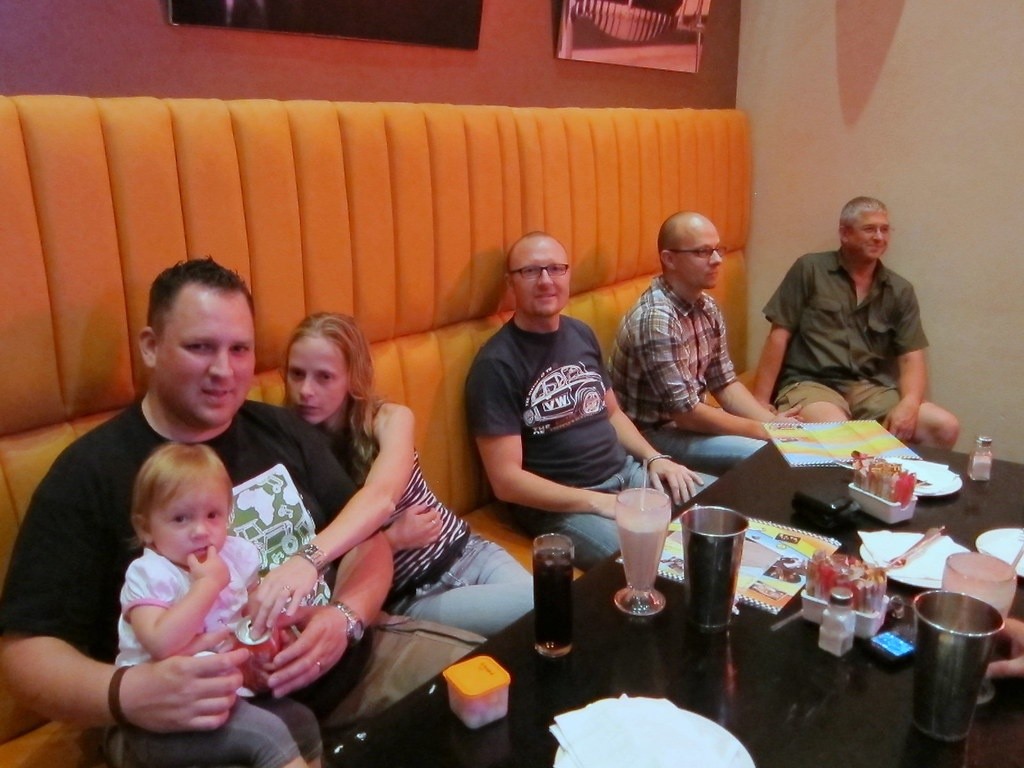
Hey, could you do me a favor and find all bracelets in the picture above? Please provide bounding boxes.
[298,544,327,572]
[108,665,131,725]
[647,455,672,466]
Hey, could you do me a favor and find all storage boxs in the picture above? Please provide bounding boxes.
[440,656,511,728]
[847,484,918,524]
[800,590,889,638]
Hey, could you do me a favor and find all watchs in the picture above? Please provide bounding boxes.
[333,602,365,639]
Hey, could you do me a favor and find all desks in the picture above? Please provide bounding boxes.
[320,444,1024,767]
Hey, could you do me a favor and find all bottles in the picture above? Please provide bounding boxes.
[967,435,994,481]
[817,588,856,656]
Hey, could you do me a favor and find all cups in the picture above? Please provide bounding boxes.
[680,506,750,631]
[612,488,672,616]
[534,532,575,656]
[942,552,1018,704]
[913,591,1005,740]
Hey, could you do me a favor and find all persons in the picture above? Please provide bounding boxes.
[245,312,533,640]
[106,445,323,768]
[463,232,718,563]
[754,196,959,448]
[2,257,488,768]
[986,617,1024,680]
[607,210,802,468]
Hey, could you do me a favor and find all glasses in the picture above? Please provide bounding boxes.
[668,246,727,258]
[509,263,570,279]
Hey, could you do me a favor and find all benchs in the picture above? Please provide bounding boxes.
[1,92,749,767]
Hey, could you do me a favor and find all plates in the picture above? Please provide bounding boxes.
[976,528,1023,576]
[860,532,973,589]
[552,708,753,767]
[887,460,964,496]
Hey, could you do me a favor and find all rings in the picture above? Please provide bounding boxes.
[282,585,289,590]
[432,520,437,525]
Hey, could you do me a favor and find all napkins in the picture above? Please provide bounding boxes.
[550,692,721,766]
[883,458,958,493]
[859,530,946,582]
